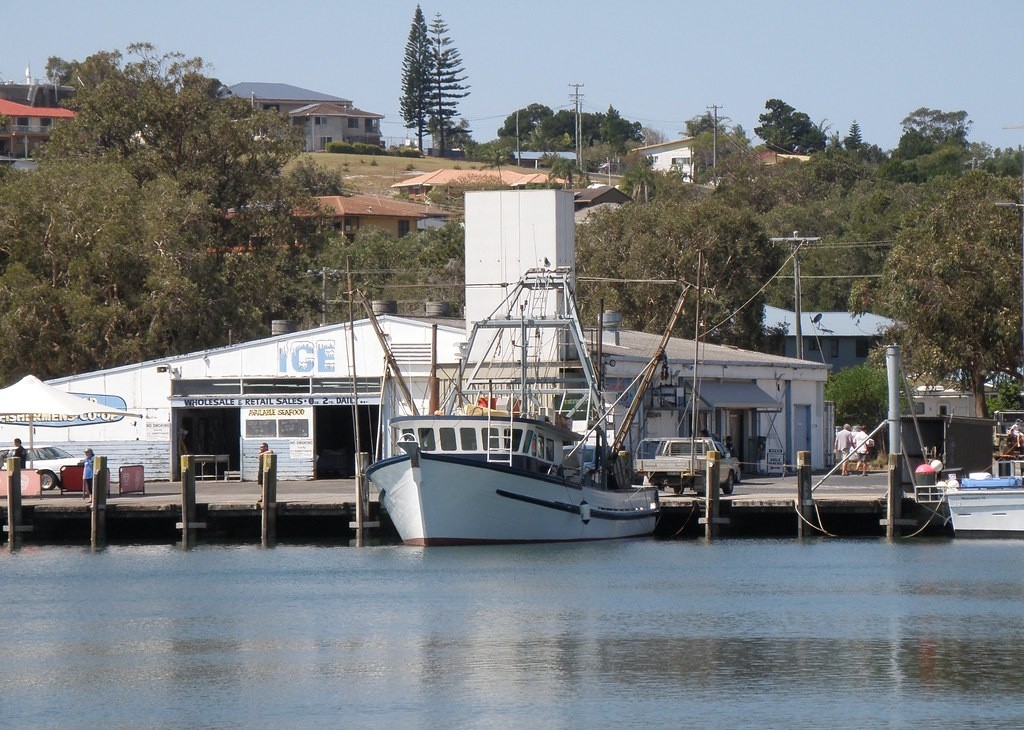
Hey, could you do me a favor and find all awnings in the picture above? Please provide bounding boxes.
[687,381,783,413]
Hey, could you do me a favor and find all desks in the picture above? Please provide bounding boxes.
[190,455,230,483]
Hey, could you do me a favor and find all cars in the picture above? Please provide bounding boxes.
[0,445,86,491]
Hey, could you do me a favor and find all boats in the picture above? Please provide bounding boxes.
[912,460,1024,538]
[363,264,660,548]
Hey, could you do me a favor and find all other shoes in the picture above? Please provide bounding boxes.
[842,472,849,476]
[862,474,868,476]
[258,499,262,502]
[1017,444,1021,447]
[86,500,92,503]
[855,468,858,471]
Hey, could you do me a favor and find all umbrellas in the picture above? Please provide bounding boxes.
[0,375,142,469]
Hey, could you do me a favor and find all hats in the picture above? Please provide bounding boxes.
[1016,419,1022,422]
[83,448,92,452]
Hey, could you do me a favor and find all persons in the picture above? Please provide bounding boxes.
[82,448,95,503]
[724,436,735,458]
[833,424,855,476]
[13,437,26,469]
[853,425,871,476]
[257,443,273,502]
[1009,419,1024,448]
[700,429,716,441]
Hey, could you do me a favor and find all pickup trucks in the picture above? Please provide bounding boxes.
[634,436,743,496]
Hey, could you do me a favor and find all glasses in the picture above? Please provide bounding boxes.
[85,452,87,453]
